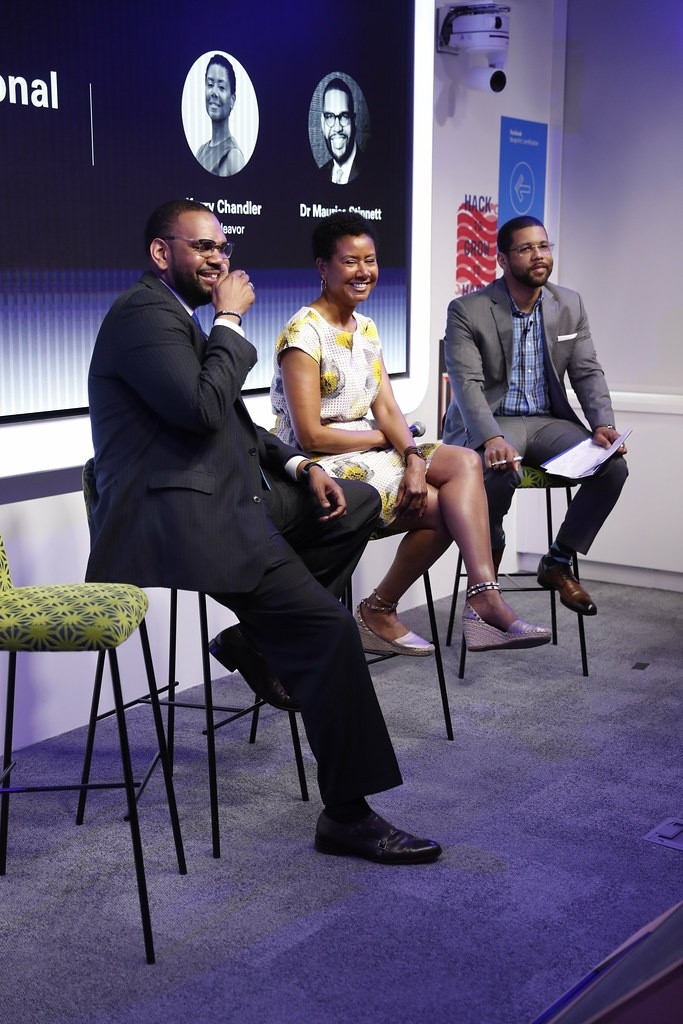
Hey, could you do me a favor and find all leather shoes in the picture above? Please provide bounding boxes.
[492,531,506,583]
[207,623,301,713]
[315,806,443,866]
[537,555,599,618]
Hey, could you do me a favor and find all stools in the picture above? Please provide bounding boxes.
[75,456,308,857]
[0,534,187,965]
[249,529,456,744]
[446,463,590,678]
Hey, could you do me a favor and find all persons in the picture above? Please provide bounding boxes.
[319,78,367,184]
[442,215,629,616]
[269,211,552,656]
[84,199,442,866]
[195,54,245,176]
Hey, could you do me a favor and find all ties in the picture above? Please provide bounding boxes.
[192,313,272,492]
[336,169,343,183]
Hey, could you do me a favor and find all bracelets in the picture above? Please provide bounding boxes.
[213,310,242,327]
[595,424,616,431]
[300,462,325,481]
[402,446,427,462]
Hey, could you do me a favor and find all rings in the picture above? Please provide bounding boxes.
[247,282,255,291]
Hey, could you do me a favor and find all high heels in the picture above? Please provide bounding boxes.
[461,581,551,652]
[354,587,438,658]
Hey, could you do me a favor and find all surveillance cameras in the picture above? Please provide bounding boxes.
[462,65,506,93]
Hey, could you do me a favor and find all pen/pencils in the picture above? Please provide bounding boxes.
[492,456,523,465]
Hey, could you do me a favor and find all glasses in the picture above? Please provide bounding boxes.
[507,241,555,256]
[163,236,235,260]
[322,111,356,128]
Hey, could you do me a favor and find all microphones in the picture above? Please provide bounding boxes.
[408,422,425,438]
